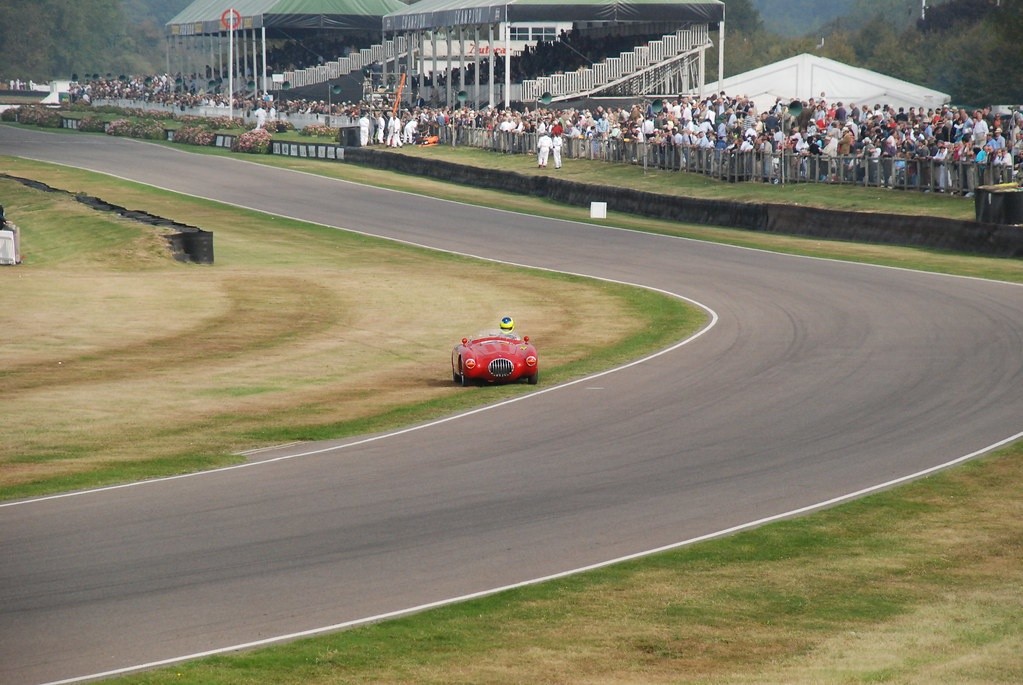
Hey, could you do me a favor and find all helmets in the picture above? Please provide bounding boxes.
[499,317,515,335]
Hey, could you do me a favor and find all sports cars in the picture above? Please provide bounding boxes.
[451,330,539,386]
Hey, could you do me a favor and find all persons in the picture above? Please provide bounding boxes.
[69,24,1023,197]
[536,131,553,167]
[498,316,515,338]
